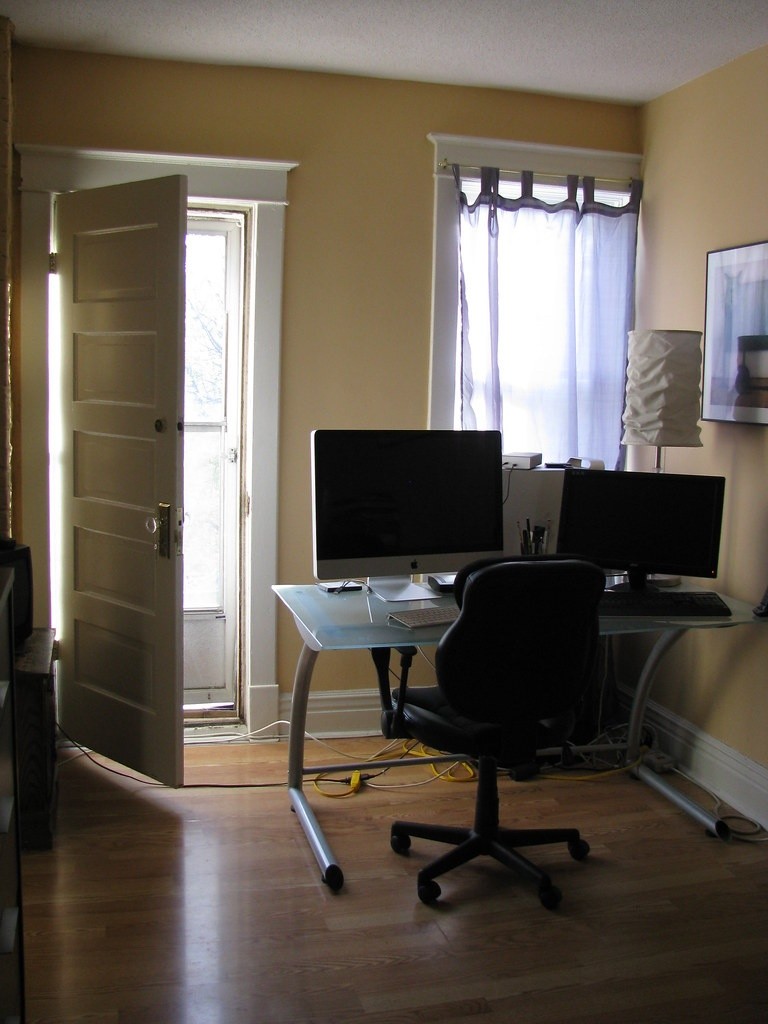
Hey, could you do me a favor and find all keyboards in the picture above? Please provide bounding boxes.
[388,607,461,628]
[596,591,733,617]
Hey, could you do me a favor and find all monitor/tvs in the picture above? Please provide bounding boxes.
[310,429,503,600]
[556,469,725,591]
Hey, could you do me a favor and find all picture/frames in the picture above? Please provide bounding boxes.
[701,239,768,427]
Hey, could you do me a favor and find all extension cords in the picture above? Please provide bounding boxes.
[642,745,673,772]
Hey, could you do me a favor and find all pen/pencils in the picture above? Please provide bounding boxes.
[515,516,544,554]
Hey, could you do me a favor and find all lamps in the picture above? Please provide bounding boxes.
[619,328,705,587]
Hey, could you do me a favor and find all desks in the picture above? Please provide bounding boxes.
[270,581,767,896]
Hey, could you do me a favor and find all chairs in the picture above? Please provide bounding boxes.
[381,560,606,912]
